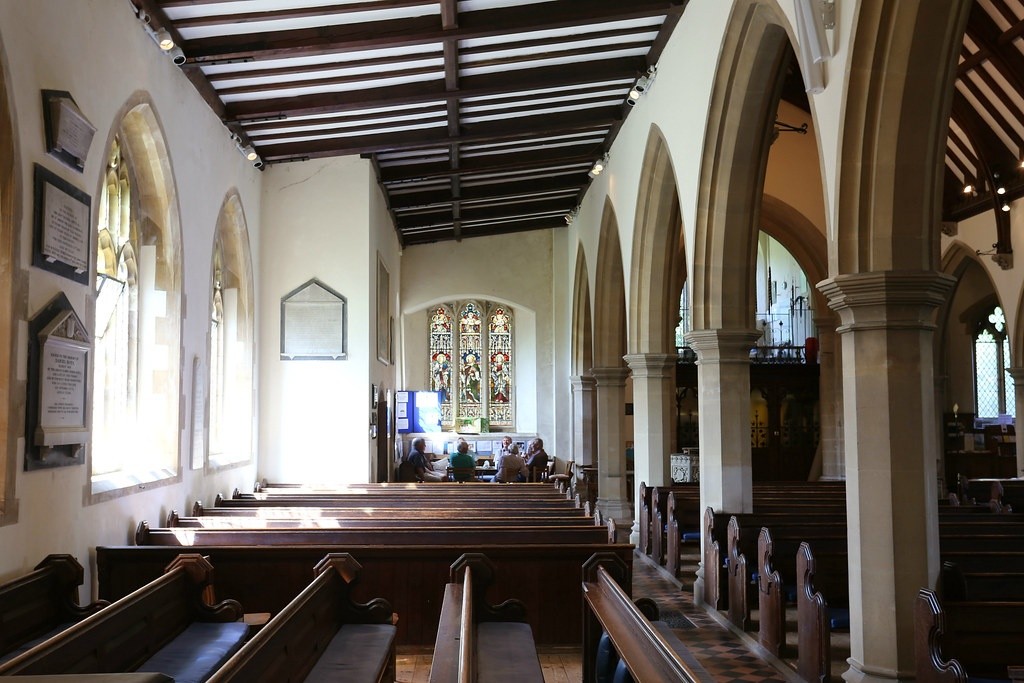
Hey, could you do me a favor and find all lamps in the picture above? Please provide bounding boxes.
[629,86,640,100]
[1002,196,1012,212]
[595,159,605,171]
[587,171,595,179]
[634,75,649,93]
[961,176,976,196]
[564,204,580,225]
[591,165,599,175]
[169,46,190,66]
[251,154,263,169]
[626,95,637,107]
[152,27,174,51]
[244,145,258,161]
[996,181,1007,195]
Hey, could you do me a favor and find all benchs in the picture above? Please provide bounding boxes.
[0,481,1024,683]
[399,453,451,481]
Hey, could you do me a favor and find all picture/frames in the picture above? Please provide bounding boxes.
[375,249,390,365]
[371,383,379,424]
[390,316,394,365]
[29,161,92,290]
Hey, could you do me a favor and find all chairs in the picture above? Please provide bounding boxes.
[502,467,519,483]
[446,466,475,480]
[534,466,550,481]
[549,460,575,496]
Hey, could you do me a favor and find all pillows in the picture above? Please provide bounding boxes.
[431,457,449,470]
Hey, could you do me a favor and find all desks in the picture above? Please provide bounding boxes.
[476,466,498,475]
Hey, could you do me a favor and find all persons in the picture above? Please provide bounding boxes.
[494,436,520,469]
[407,437,449,482]
[519,438,548,482]
[490,444,530,483]
[448,437,483,482]
